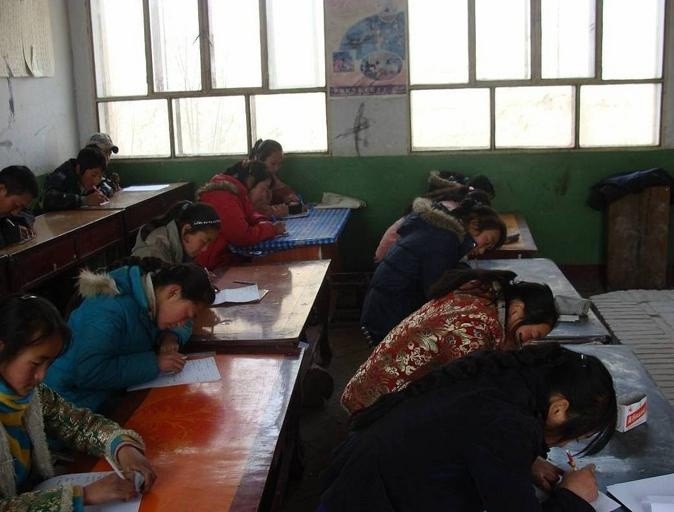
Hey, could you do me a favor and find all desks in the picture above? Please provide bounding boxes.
[0,209,124,298]
[471,257,611,345]
[229,202,352,322]
[535,345,674,511]
[189,260,333,469]
[494,212,539,259]
[88,344,314,512]
[77,182,194,254]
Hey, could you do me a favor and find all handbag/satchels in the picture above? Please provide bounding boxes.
[587,168,670,211]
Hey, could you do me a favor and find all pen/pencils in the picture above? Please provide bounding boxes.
[272,215,276,222]
[566,450,576,472]
[106,457,138,498]
[205,267,210,276]
[6,218,26,241]
[233,280,256,285]
[519,332,523,350]
[92,185,109,201]
[475,258,480,269]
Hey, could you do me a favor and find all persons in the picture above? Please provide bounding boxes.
[88,132,122,194]
[0,165,39,249]
[40,256,216,415]
[44,145,114,212]
[313,341,615,512]
[127,199,221,283]
[1,293,157,512]
[337,268,560,416]
[246,138,306,218]
[357,174,506,346]
[187,160,277,268]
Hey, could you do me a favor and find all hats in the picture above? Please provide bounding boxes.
[86,133,118,153]
